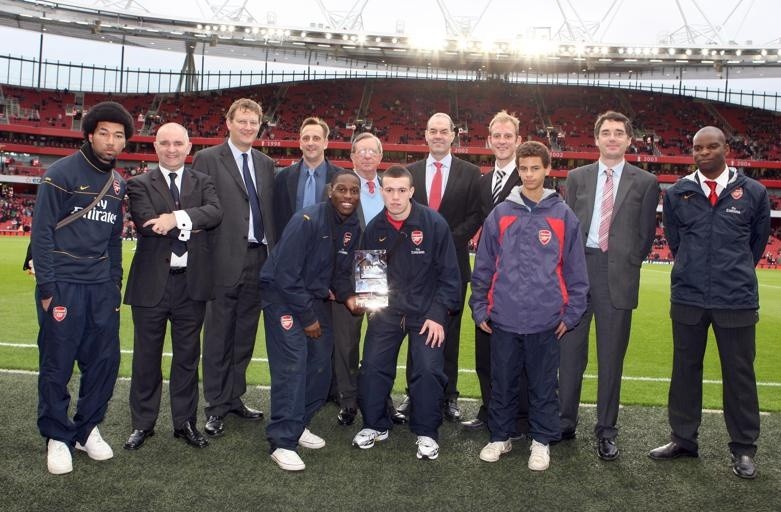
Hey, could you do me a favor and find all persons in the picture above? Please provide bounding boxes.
[649,125,770,477]
[190,98,277,438]
[460,110,557,441]
[123,122,224,450]
[31,100,135,475]
[548,110,663,462]
[467,141,591,471]
[258,170,361,471]
[276,117,365,402]
[397,112,482,422]
[1,68,780,274]
[351,167,462,460]
[332,133,408,425]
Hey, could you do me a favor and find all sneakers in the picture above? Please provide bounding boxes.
[480,439,512,462]
[48,426,113,474]
[528,439,550,471]
[270,427,325,471]
[339,408,439,460]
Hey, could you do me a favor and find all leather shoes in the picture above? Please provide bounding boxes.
[648,442,698,460]
[731,455,756,478]
[123,429,154,450]
[598,439,619,461]
[444,402,485,430]
[174,405,263,448]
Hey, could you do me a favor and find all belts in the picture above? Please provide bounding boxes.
[170,267,187,274]
[247,243,265,247]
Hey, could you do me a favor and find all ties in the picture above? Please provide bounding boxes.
[599,170,614,253]
[302,170,316,207]
[704,181,718,205]
[367,182,375,193]
[168,173,188,257]
[492,171,506,205]
[429,162,443,211]
[242,154,264,242]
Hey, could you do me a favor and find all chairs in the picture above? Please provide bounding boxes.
[1,64,781,272]
